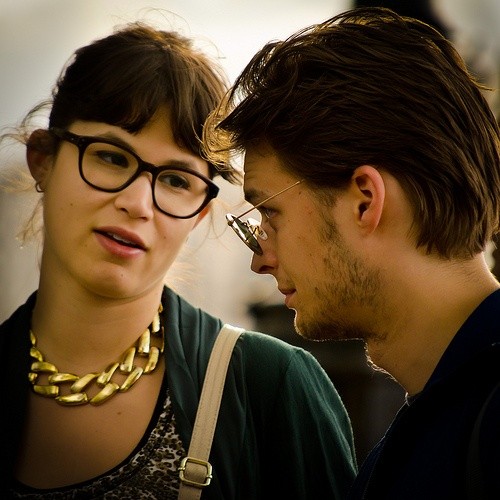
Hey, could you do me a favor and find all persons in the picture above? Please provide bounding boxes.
[0,25,360,500]
[216,7,500,500]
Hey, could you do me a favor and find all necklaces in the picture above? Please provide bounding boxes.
[27,297,163,407]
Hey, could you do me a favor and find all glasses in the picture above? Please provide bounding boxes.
[225,176,311,256]
[47,123,221,221]
